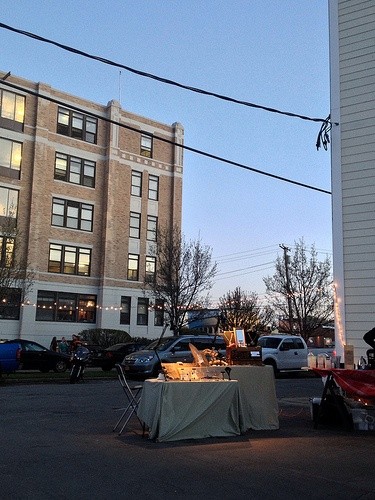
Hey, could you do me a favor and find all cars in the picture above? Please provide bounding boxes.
[97,342,146,372]
[2,339,72,373]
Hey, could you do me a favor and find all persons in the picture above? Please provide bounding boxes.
[363,327,375,349]
[72,335,80,346]
[60,337,69,353]
[50,337,57,352]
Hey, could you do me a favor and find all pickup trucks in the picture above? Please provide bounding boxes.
[0,343,22,373]
[251,335,335,376]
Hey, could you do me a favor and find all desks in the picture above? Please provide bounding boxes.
[300,366,375,429]
[135,377,246,445]
[159,363,281,434]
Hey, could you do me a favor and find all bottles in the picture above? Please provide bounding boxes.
[308,351,314,367]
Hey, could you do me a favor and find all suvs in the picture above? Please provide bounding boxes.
[77,328,137,367]
[121,334,228,380]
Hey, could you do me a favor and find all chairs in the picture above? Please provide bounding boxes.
[112,364,141,435]
